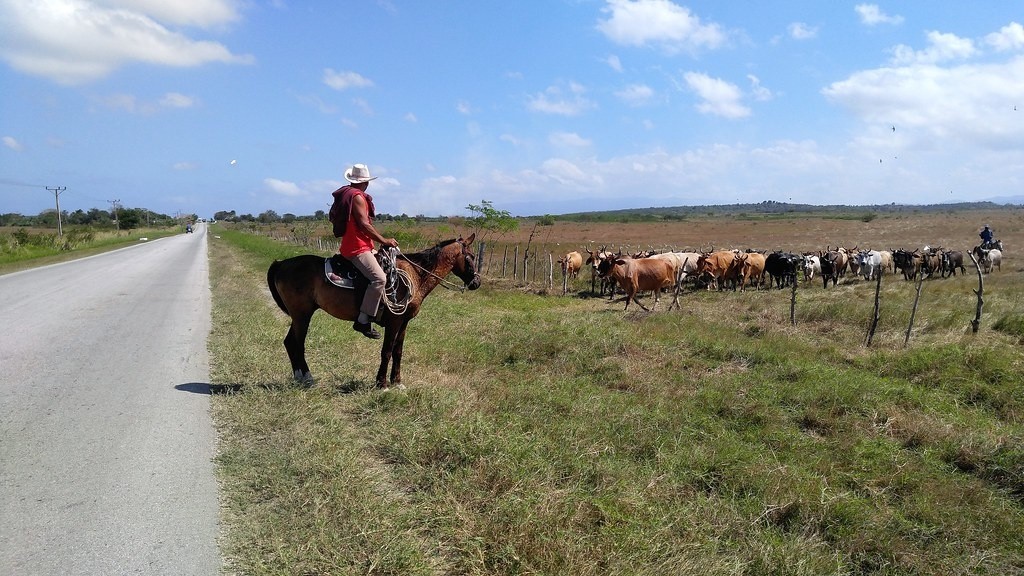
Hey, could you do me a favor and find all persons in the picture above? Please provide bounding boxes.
[186,221,191,230]
[981,225,993,243]
[339,164,400,339]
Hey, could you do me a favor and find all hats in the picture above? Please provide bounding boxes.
[344,164,378,184]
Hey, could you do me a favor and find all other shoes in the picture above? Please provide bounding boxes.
[353,320,382,339]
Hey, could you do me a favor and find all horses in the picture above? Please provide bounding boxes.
[973,240,1004,265]
[266,232,481,392]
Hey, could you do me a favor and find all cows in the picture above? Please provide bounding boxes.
[557,251,583,279]
[696,244,821,293]
[818,245,892,290]
[890,247,966,283]
[598,245,682,312]
[585,244,702,299]
[977,246,1002,274]
